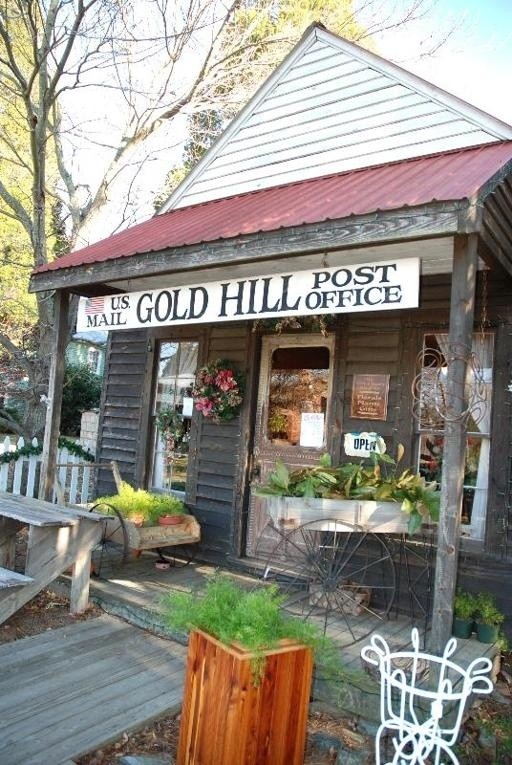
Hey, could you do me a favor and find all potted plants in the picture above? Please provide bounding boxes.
[246,432,442,537]
[154,563,373,764]
[144,492,191,528]
[451,583,479,639]
[92,480,159,529]
[474,591,503,644]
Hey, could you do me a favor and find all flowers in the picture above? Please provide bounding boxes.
[250,310,340,340]
[189,357,247,426]
[150,405,187,467]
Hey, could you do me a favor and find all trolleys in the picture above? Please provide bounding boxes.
[47,460,204,583]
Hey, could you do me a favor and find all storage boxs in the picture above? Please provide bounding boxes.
[307,577,372,617]
